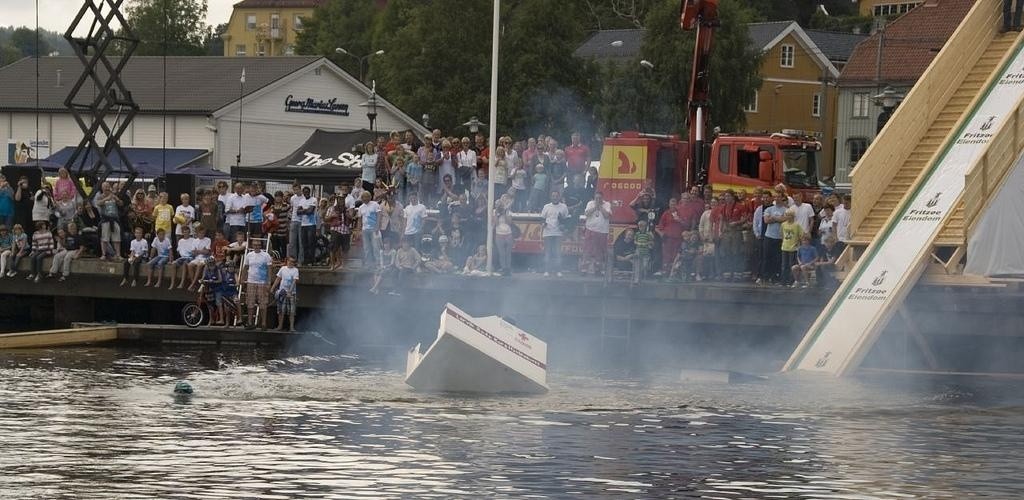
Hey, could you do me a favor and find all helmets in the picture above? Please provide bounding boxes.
[204,257,218,264]
[226,261,236,267]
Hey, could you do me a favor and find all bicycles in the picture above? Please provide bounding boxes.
[180,277,261,329]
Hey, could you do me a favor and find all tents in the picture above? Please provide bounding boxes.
[231,129,424,193]
[31,147,210,181]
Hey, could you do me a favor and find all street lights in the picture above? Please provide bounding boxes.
[421,114,429,129]
[360,82,386,131]
[870,87,903,122]
[333,45,384,84]
[463,116,488,146]
[237,67,246,164]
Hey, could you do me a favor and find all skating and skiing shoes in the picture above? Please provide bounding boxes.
[245,324,296,333]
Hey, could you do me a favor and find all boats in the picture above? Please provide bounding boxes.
[404,302,552,399]
[0,322,119,351]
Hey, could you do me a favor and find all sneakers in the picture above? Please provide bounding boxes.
[669,267,822,291]
[543,271,563,278]
[5,270,17,279]
[45,273,56,279]
[24,274,43,283]
[57,277,68,284]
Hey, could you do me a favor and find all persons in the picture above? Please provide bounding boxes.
[492,134,612,277]
[0,166,354,291]
[236,240,272,330]
[614,181,852,291]
[222,260,241,327]
[270,255,299,332]
[197,253,223,325]
[351,129,490,292]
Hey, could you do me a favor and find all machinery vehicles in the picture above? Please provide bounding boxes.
[415,0,833,270]
